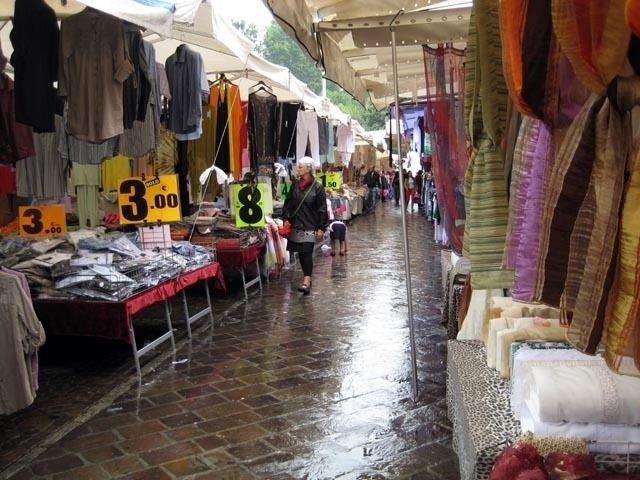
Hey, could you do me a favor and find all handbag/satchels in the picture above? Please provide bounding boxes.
[279,219,295,238]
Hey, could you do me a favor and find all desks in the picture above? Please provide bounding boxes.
[215,242,266,299]
[442,336,639,479]
[31,258,222,373]
[442,248,468,339]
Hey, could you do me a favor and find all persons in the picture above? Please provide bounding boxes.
[353,163,425,218]
[327,220,349,256]
[282,156,327,291]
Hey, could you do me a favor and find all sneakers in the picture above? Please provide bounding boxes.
[331,253,335,256]
[298,284,311,294]
[340,253,344,255]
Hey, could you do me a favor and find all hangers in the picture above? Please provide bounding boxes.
[249,85,276,96]
[174,33,190,53]
[247,77,276,89]
[208,71,239,88]
